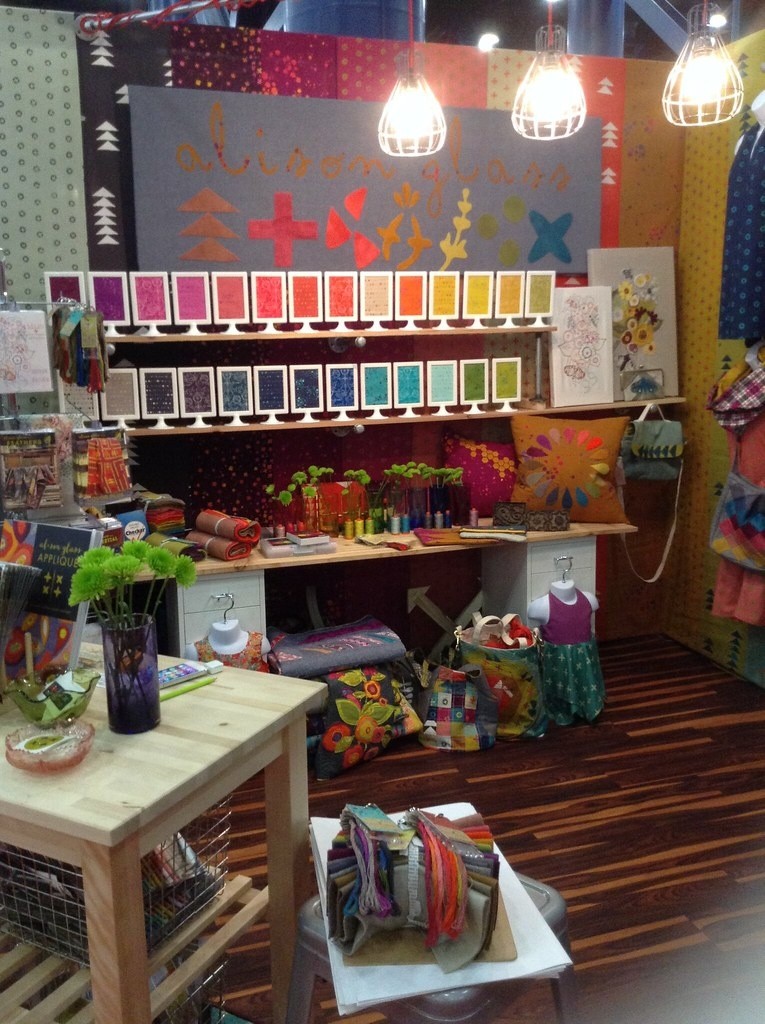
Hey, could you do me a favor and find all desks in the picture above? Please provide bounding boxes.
[133,518,638,663]
[0,642,328,1024]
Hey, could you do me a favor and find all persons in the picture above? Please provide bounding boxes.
[194,620,274,673]
[704,343,765,625]
[529,579,602,727]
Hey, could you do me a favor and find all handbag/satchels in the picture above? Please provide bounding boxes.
[412,657,498,751]
[615,402,685,583]
[454,612,548,740]
[298,480,369,537]
[708,435,765,574]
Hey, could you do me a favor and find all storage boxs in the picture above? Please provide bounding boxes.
[260,530,337,558]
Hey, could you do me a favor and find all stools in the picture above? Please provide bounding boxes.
[287,871,581,1024]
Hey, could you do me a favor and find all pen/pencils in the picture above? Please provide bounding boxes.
[161,676,217,701]
[23,630,36,686]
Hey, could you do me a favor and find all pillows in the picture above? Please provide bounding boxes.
[510,413,630,524]
[442,427,520,517]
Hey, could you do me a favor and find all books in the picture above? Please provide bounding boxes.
[0,519,102,704]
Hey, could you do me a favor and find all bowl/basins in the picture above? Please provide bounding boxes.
[5,719,95,773]
[2,664,102,729]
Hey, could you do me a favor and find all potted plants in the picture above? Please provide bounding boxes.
[265,460,464,538]
[68,540,198,734]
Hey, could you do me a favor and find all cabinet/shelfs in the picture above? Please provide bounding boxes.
[105,326,685,437]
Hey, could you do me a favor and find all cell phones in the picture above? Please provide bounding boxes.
[158,662,207,689]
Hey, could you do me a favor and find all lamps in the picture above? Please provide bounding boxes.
[510,0,586,142]
[661,0,745,127]
[377,0,448,158]
